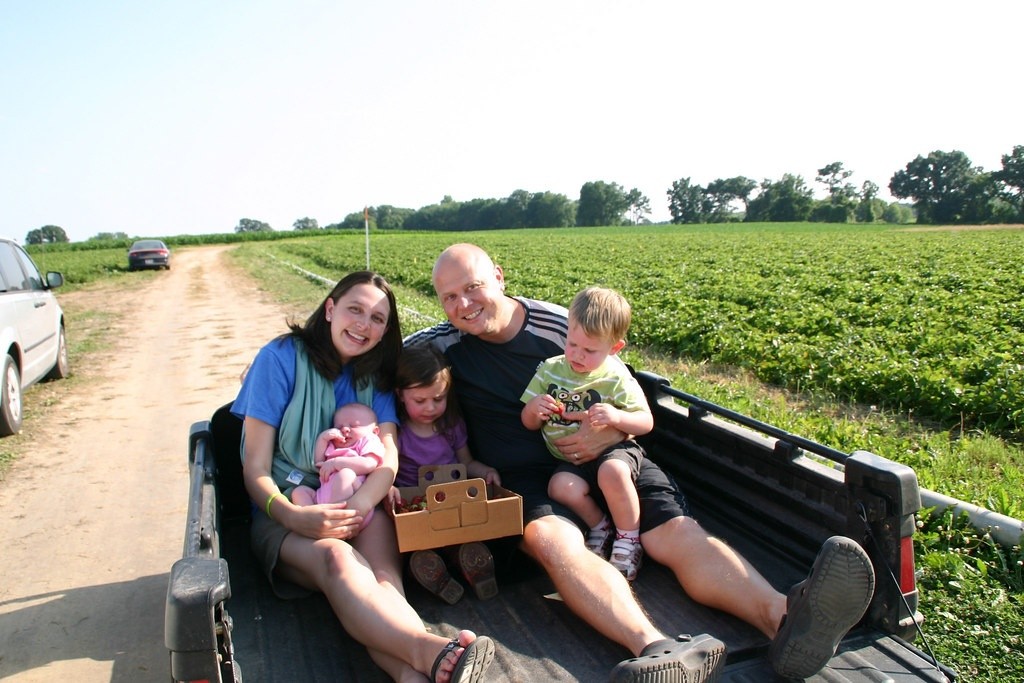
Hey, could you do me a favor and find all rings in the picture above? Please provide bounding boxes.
[573,452,582,460]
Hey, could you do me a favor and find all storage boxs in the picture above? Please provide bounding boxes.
[392,464,523,553]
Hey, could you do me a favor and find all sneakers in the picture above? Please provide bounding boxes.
[584,523,616,562]
[608,538,644,581]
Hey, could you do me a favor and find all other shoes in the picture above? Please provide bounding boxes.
[456,541,500,602]
[409,550,465,606]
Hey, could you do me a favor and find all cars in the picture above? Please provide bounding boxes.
[125,239,171,272]
[0,238,69,438]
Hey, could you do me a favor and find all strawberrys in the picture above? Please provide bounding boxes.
[395,491,502,514]
[552,402,565,414]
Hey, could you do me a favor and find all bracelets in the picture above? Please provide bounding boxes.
[266,493,290,521]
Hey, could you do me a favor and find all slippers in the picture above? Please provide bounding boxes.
[430,637,495,683]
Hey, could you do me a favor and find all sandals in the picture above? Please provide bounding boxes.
[767,535,876,680]
[608,632,729,683]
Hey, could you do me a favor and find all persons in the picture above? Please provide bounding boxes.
[290,400,385,538]
[229,271,496,683]
[401,242,876,683]
[381,342,502,604]
[519,286,654,582]
